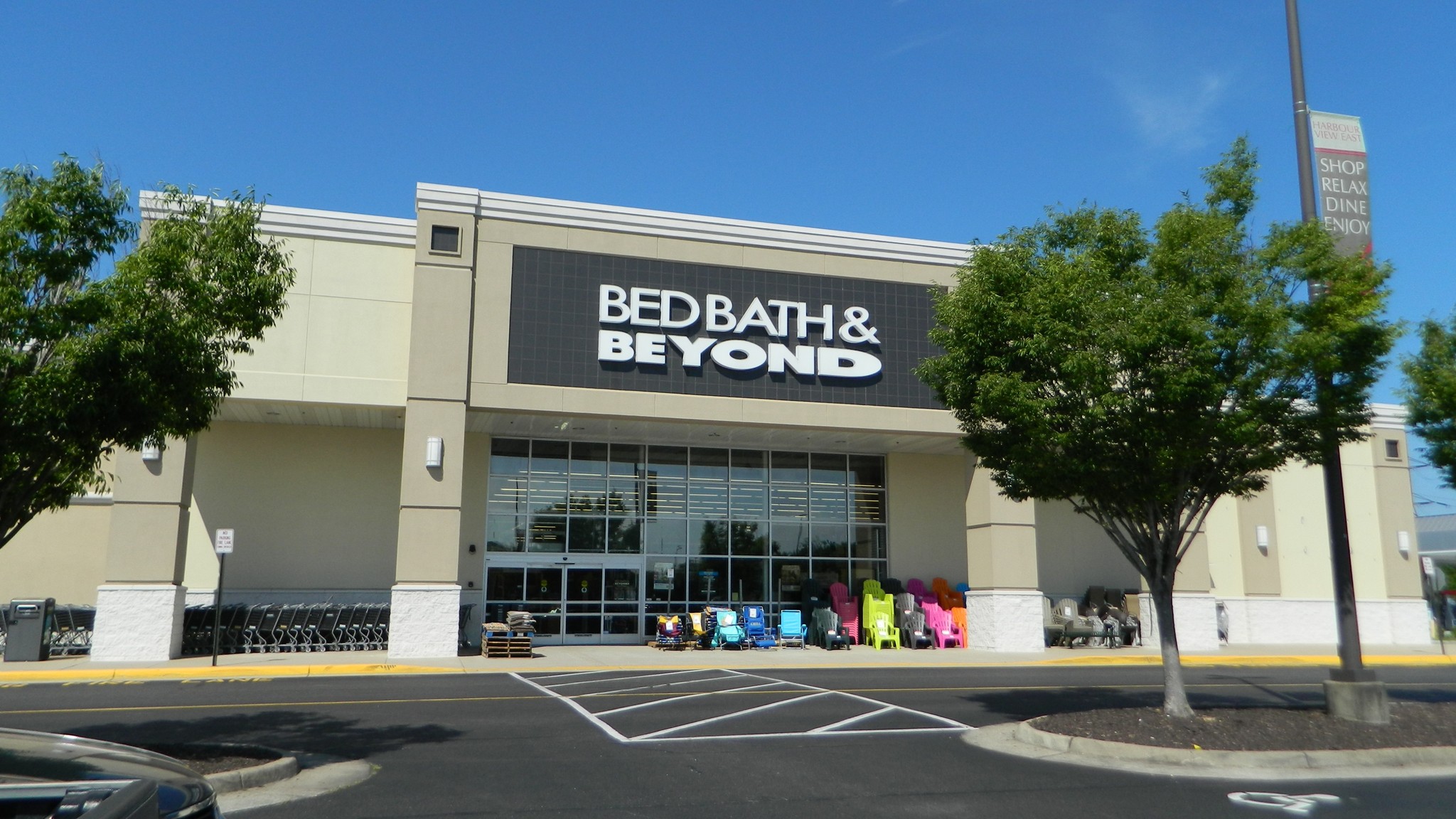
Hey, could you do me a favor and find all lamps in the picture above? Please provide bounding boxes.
[142,435,160,459]
[1256,526,1269,546]
[1398,531,1409,553]
[468,545,477,555]
[425,436,443,468]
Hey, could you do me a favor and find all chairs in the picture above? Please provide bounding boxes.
[1043,587,1141,648]
[1216,602,1230,644]
[656,578,971,651]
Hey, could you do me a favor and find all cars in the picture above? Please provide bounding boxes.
[0,724,227,819]
